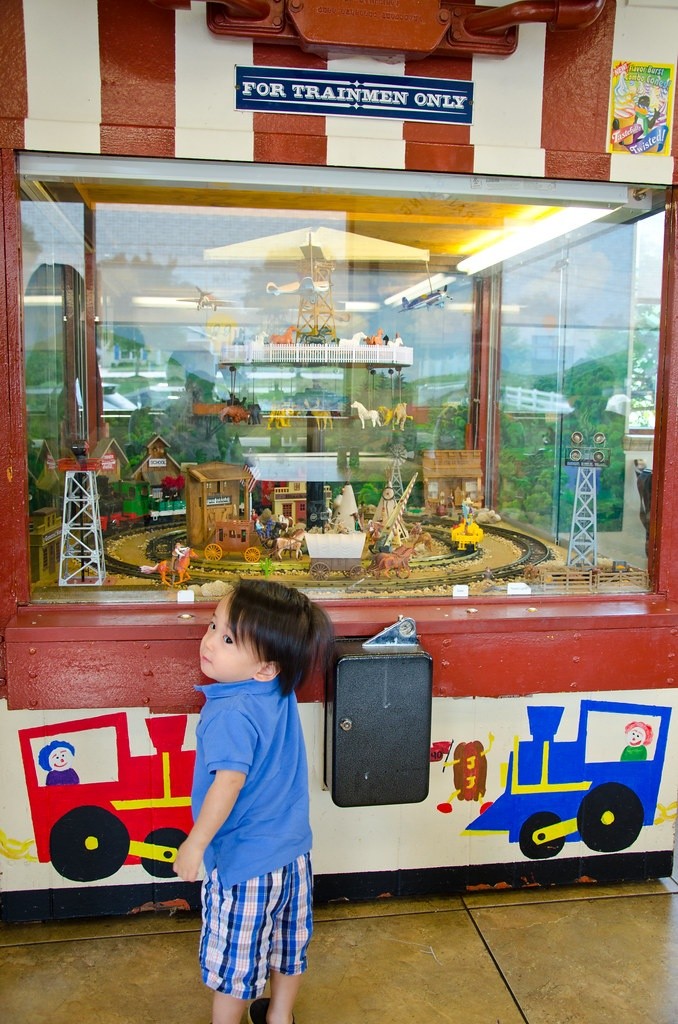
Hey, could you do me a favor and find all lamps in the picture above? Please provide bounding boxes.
[383,207,620,307]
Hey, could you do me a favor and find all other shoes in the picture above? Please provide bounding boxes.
[248,998,295,1024]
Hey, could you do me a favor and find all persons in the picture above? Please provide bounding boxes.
[431,346,525,451]
[171,579,335,1024]
[546,357,625,444]
[25,263,105,438]
[125,344,247,465]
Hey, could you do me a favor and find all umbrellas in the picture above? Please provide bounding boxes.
[200,223,433,337]
